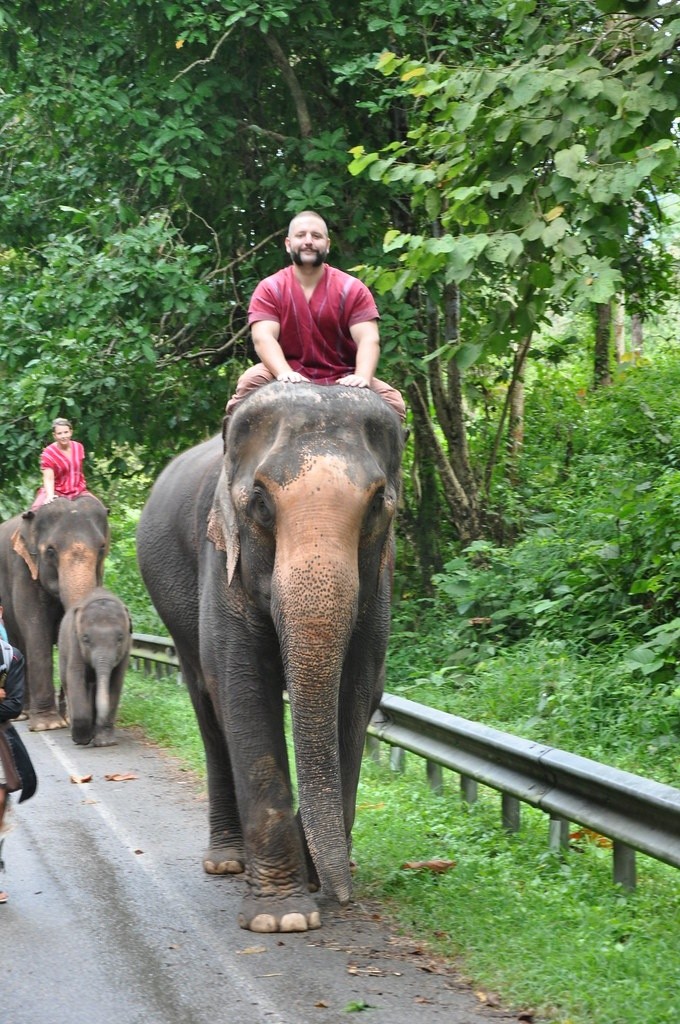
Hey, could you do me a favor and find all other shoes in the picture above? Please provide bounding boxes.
[0,890,9,902]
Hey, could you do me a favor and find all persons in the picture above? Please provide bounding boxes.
[0,596,36,903]
[225,210,406,424]
[32,418,110,515]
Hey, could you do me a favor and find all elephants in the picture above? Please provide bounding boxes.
[0,493,134,749]
[136,383,410,936]
